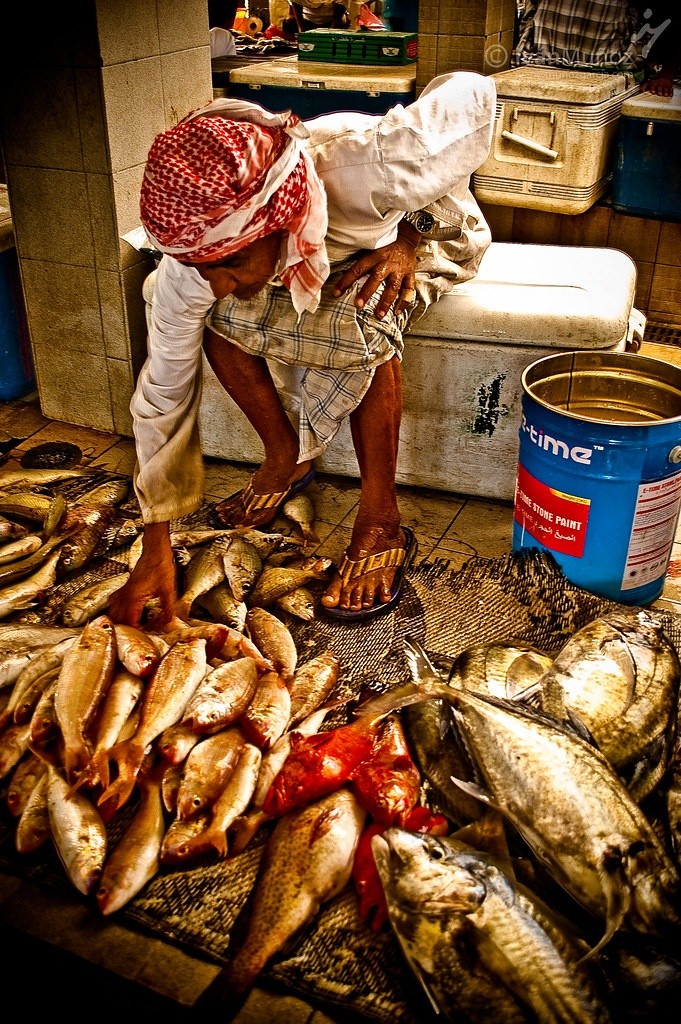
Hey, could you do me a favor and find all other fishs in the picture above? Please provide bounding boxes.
[1,464,681,1024]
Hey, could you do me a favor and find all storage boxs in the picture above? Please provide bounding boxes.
[197,245,648,500]
[0,183,36,401]
[611,75,681,224]
[224,61,416,121]
[295,27,419,66]
[471,64,646,217]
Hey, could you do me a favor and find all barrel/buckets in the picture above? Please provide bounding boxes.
[516,350,681,605]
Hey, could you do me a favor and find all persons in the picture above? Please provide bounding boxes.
[109,71,498,630]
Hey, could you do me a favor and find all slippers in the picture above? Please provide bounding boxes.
[206,461,316,532]
[319,526,419,624]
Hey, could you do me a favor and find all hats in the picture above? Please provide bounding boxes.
[139,96,330,324]
